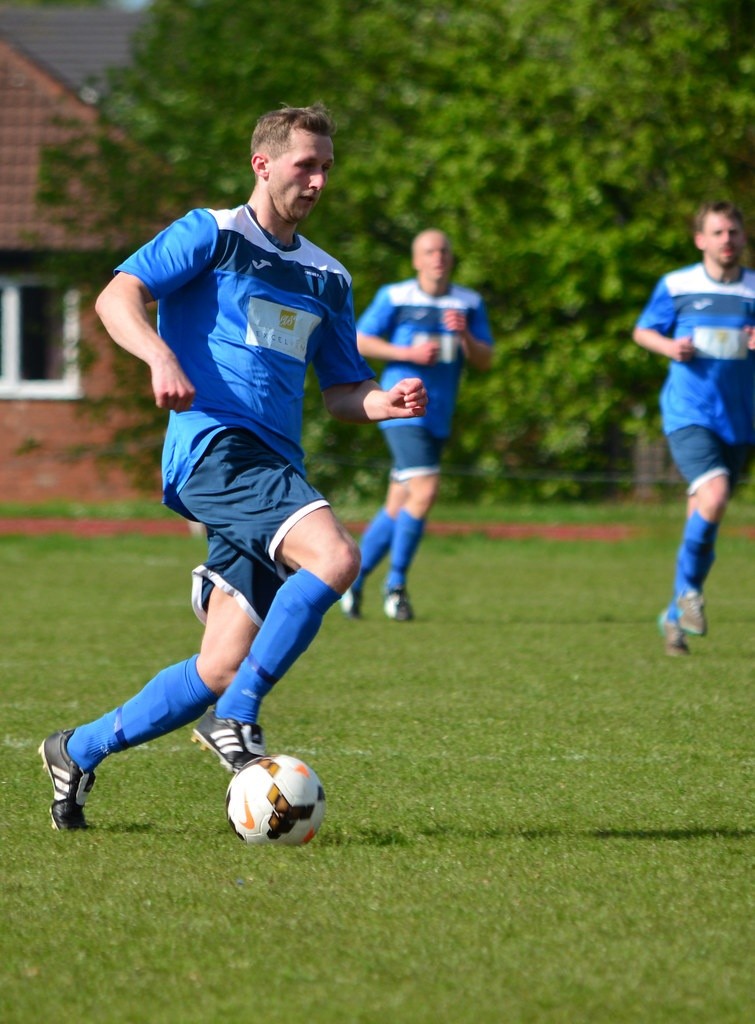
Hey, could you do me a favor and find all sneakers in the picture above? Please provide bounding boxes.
[381,589,413,619]
[676,587,709,637]
[341,586,360,622]
[191,708,264,775]
[37,727,96,831]
[661,605,692,659]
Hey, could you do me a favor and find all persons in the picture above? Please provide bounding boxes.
[633,199,755,656]
[39,105,429,830]
[338,228,498,621]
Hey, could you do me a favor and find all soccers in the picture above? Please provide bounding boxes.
[225,754,327,847]
